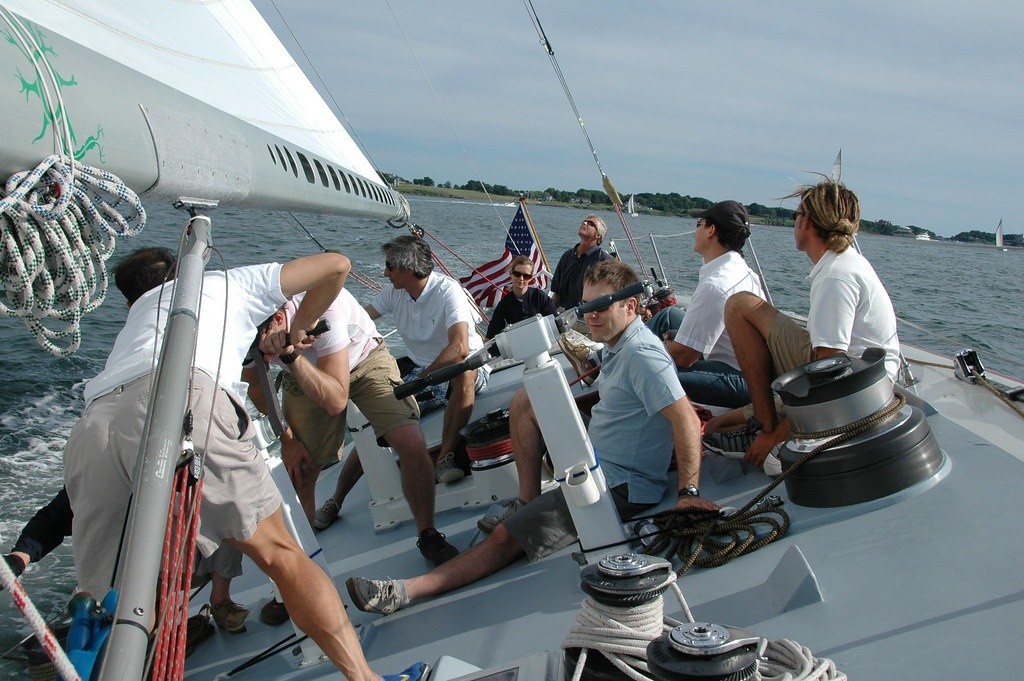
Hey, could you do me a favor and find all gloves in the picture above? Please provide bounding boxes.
[0,555,26,589]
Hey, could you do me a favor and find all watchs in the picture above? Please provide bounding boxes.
[278,348,301,365]
[676,485,702,497]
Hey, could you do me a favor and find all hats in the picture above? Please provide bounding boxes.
[690,200,750,237]
[583,214,607,247]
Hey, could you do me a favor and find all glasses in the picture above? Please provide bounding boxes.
[579,301,609,312]
[512,271,532,280]
[697,221,706,228]
[386,261,395,271]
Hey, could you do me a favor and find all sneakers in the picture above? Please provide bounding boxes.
[416,527,459,567]
[378,662,431,680]
[314,497,342,528]
[701,416,763,459]
[477,498,524,533]
[558,333,600,385]
[210,598,249,631]
[346,576,401,614]
[261,597,289,626]
[434,452,464,483]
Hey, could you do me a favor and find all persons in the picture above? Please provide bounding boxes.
[479,255,563,359]
[556,200,777,410]
[346,260,722,616]
[59,244,431,681]
[295,234,493,531]
[1,486,251,634]
[255,279,459,572]
[550,215,620,310]
[700,169,902,467]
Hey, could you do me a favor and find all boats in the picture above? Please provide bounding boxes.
[915,232,930,240]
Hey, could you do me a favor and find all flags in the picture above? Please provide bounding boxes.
[458,206,549,309]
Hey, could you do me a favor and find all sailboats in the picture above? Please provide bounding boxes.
[628,193,638,218]
[995,218,1008,251]
